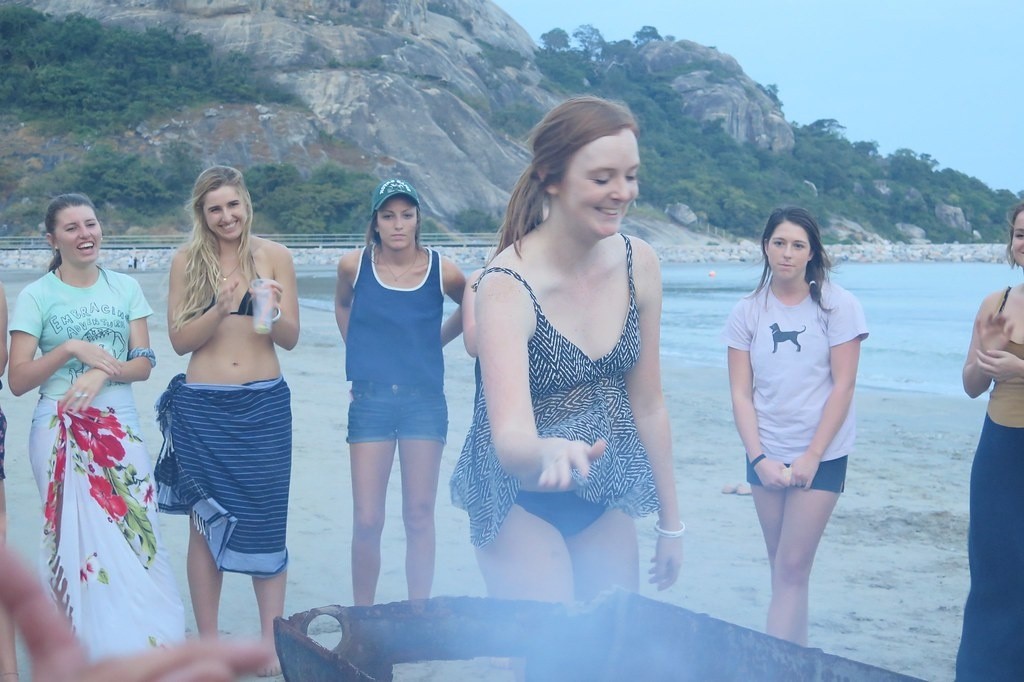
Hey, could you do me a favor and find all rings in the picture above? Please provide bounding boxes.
[76,391,80,397]
[83,394,88,397]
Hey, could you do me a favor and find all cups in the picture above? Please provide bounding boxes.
[250,278,283,335]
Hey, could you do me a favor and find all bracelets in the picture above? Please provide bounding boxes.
[1,672,18,675]
[654,520,685,538]
[751,455,766,467]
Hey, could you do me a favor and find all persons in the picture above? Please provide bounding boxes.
[461,198,549,415]
[447,96,684,602]
[8,194,186,663]
[335,178,466,606]
[720,205,870,647]
[954,203,1024,682]
[128,255,146,269]
[0,282,8,531]
[154,166,300,677]
[0,540,273,682]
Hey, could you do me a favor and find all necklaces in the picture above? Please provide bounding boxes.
[220,263,239,281]
[383,254,417,281]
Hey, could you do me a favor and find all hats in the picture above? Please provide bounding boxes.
[370,176,420,216]
